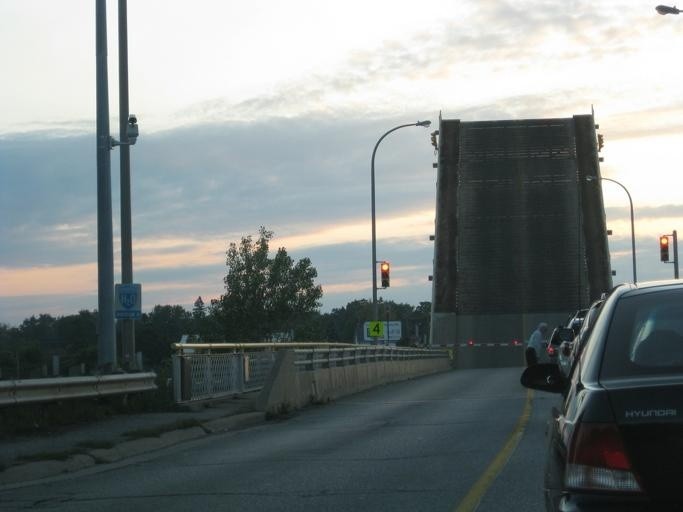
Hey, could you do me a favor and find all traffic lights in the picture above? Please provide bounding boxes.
[660,237,669,261]
[381,264,390,287]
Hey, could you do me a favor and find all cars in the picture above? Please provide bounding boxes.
[520,279,682,511]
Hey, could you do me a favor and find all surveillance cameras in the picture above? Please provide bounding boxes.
[128,114,137,123]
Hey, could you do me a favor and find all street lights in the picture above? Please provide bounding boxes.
[585,174,637,283]
[371,120,432,344]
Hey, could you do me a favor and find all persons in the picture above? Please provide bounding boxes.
[523,321,547,369]
